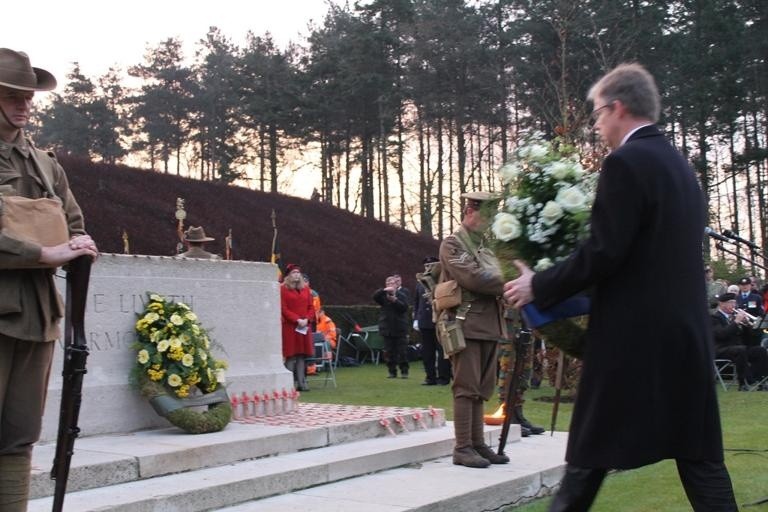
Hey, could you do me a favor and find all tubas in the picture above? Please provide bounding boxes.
[732,308,763,331]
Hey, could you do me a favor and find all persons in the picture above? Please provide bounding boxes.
[280,264,314,391]
[0,47,100,512]
[316,308,336,362]
[703,265,767,391]
[439,192,512,467]
[412,256,451,385]
[372,277,410,378]
[503,63,738,512]
[303,275,320,375]
[176,226,221,259]
[393,274,410,298]
[497,299,545,436]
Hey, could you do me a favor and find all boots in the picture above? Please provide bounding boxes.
[510,407,531,437]
[514,406,545,434]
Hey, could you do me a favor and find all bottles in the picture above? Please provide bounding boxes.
[230,392,244,420]
[283,387,291,415]
[273,387,281,417]
[243,391,256,419]
[291,385,302,413]
[263,387,276,418]
[252,388,266,418]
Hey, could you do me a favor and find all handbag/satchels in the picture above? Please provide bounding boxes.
[435,280,462,311]
[1,196,69,269]
[439,321,467,356]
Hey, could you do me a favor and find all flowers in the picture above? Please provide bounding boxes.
[492,135,602,361]
[131,294,229,432]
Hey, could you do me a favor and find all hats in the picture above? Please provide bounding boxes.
[719,293,737,301]
[0,48,57,91]
[739,278,751,285]
[286,264,303,276]
[423,257,440,266]
[185,226,215,242]
[462,192,505,215]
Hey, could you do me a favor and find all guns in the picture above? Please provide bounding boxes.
[49,253,92,507]
[499,327,530,456]
[550,350,564,438]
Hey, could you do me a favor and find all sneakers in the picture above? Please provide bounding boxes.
[422,378,437,385]
[453,449,490,468]
[473,445,509,464]
[436,378,450,385]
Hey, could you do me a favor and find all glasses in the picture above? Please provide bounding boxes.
[591,105,611,120]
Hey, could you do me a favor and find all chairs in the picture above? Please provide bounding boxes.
[304,325,384,385]
[714,359,768,391]
